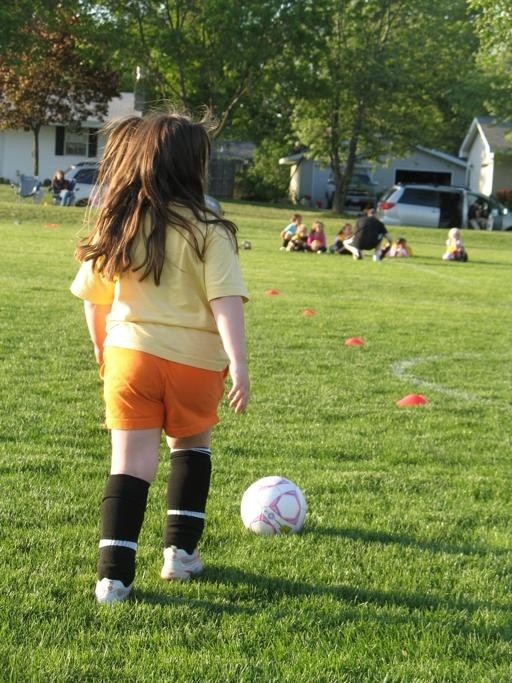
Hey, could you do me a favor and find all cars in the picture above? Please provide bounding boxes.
[203,193,225,216]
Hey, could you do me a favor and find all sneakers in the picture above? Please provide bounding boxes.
[160,544,204,580]
[93,576,134,604]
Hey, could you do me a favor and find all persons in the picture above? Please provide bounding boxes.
[442,227,468,262]
[280,208,412,261]
[53,170,74,206]
[68,113,252,606]
[469,198,486,230]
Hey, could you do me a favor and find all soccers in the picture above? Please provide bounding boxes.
[240,475,306,536]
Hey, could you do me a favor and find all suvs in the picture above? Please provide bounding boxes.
[51,161,109,206]
[374,182,511,231]
[324,172,379,213]
